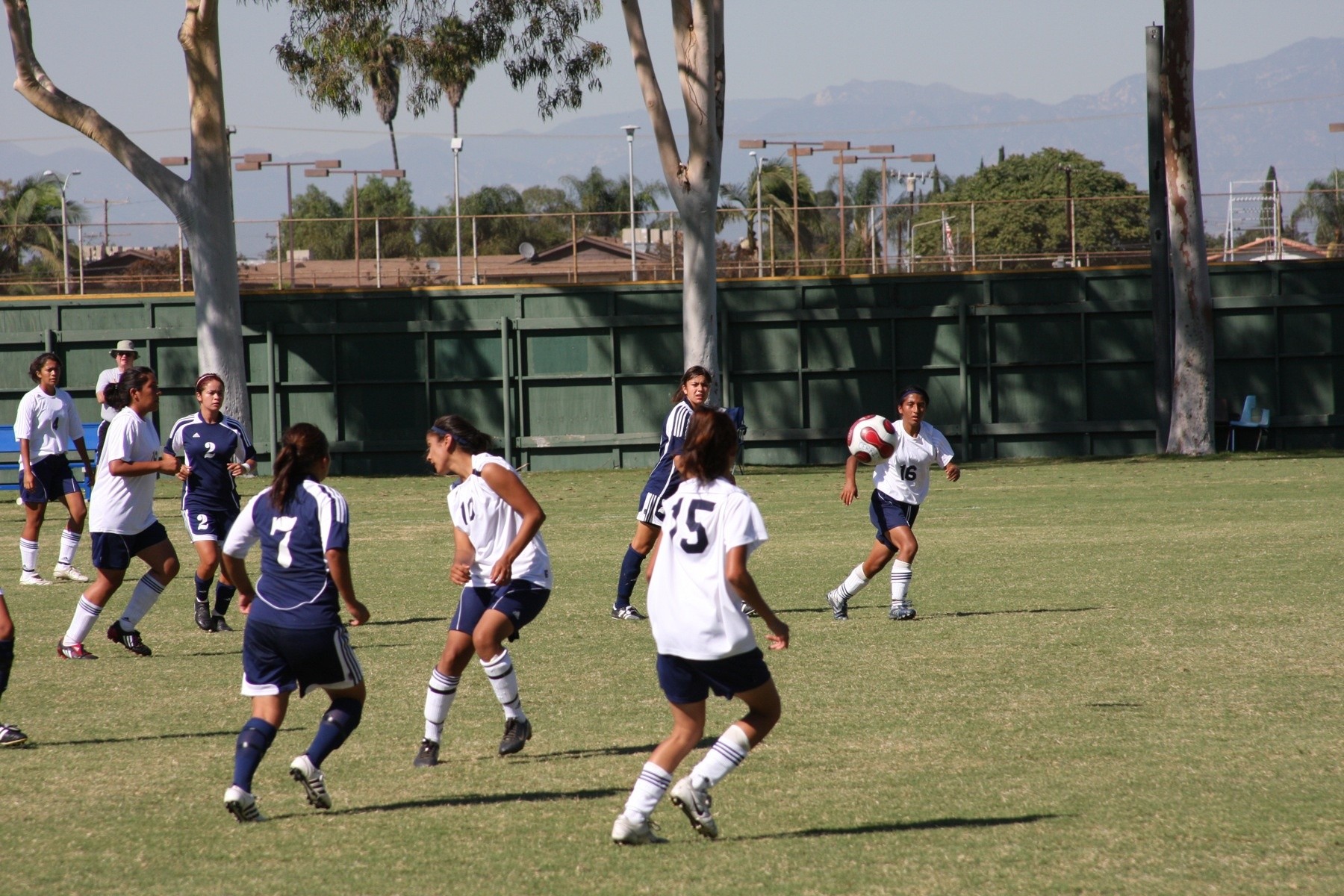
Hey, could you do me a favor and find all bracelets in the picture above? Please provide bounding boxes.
[241,463,251,472]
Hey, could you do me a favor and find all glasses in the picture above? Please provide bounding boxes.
[117,351,134,357]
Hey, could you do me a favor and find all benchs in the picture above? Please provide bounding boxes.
[0,423,100,501]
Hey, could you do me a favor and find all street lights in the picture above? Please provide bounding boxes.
[450,137,465,285]
[787,144,895,276]
[748,151,769,278]
[43,169,82,295]
[622,123,643,280]
[302,168,406,288]
[235,159,342,288]
[832,153,934,274]
[738,139,851,276]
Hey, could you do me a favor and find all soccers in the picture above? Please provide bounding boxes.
[847,414,898,463]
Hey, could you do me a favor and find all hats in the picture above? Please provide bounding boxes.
[110,340,138,360]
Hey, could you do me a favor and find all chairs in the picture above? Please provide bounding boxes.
[1227,394,1270,452]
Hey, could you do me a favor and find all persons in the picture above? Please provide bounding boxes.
[0,585,27,748]
[825,387,961,622]
[613,408,790,846]
[223,421,370,822]
[162,373,258,633]
[608,365,759,618]
[95,340,142,472]
[412,415,552,765]
[57,366,182,659]
[12,353,95,585]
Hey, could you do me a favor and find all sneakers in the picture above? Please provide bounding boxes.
[0,723,27,745]
[888,600,916,619]
[612,814,671,844]
[610,603,646,621]
[223,788,264,822]
[211,615,234,632]
[414,737,440,768]
[741,601,755,614]
[58,637,99,659]
[499,717,532,754]
[53,563,88,581]
[290,756,332,810]
[668,776,719,837]
[107,620,152,656]
[194,597,212,632]
[19,571,53,585]
[826,589,849,620]
